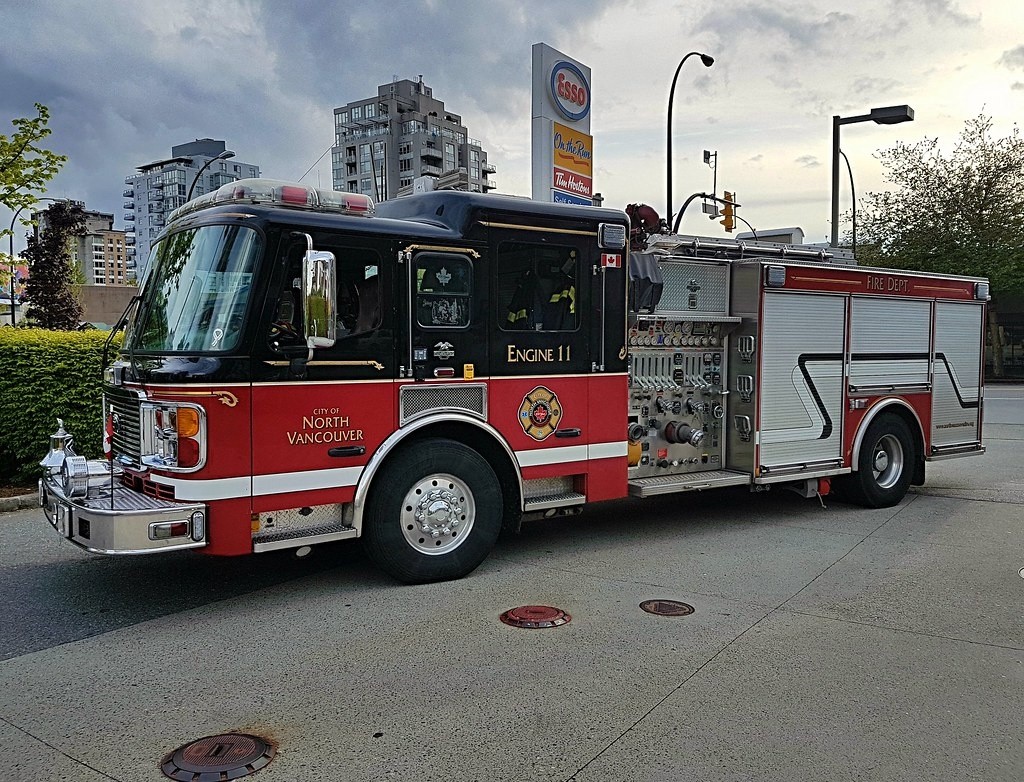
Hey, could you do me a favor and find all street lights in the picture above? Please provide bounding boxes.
[8,197,70,328]
[829,104,916,249]
[664,52,741,232]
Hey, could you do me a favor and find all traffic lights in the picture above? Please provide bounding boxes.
[718,191,734,233]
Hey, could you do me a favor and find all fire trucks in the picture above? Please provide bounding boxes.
[36,172,991,587]
